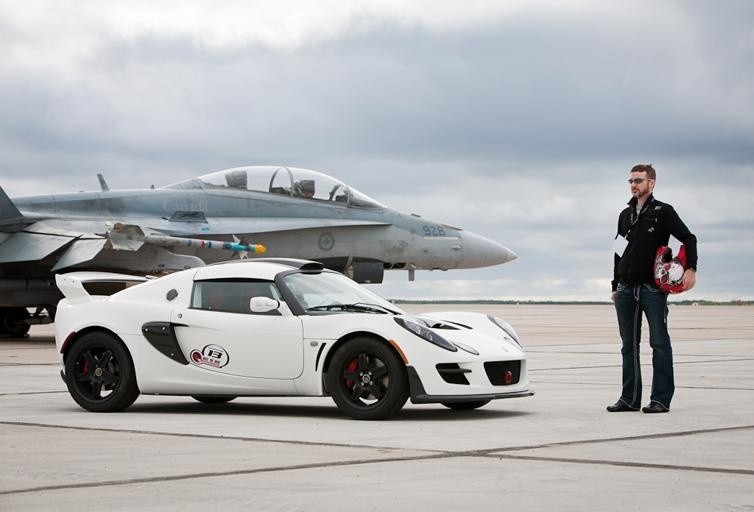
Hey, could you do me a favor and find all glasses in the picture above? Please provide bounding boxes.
[628,178,651,184]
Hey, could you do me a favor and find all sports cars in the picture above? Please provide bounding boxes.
[51,256,534,418]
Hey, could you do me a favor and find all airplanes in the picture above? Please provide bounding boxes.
[0,166,518,339]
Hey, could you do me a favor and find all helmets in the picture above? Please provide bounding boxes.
[654,245,688,294]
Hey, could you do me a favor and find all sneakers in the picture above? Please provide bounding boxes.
[606,400,641,412]
[643,400,669,413]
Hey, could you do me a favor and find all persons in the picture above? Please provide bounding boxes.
[607,163,698,413]
